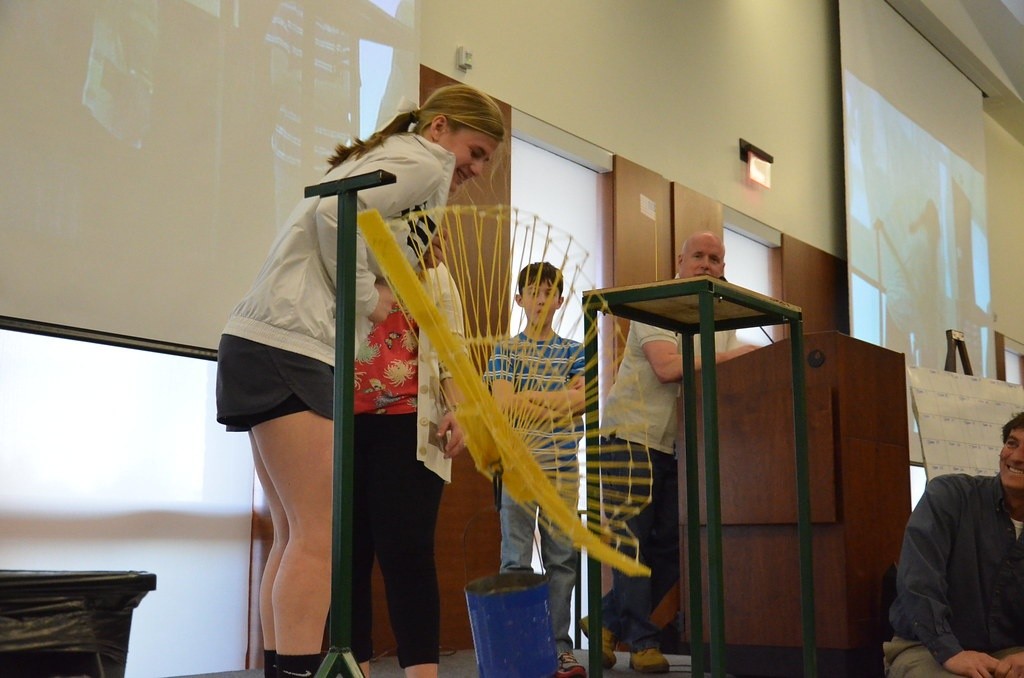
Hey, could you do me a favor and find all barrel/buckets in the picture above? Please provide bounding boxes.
[465,570,558,678]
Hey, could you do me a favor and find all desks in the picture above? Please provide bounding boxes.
[580,284,818,678]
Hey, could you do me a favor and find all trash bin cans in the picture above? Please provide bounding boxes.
[0,568,158,678]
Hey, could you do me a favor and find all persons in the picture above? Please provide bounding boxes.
[216,83,502,677]
[883,412,1024,678]
[348,261,468,678]
[484,262,586,677]
[579,231,764,671]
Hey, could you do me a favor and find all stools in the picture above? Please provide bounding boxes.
[0,569,157,678]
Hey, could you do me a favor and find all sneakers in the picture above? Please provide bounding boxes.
[555,651,587,678]
[578,615,624,669]
[629,647,671,673]
